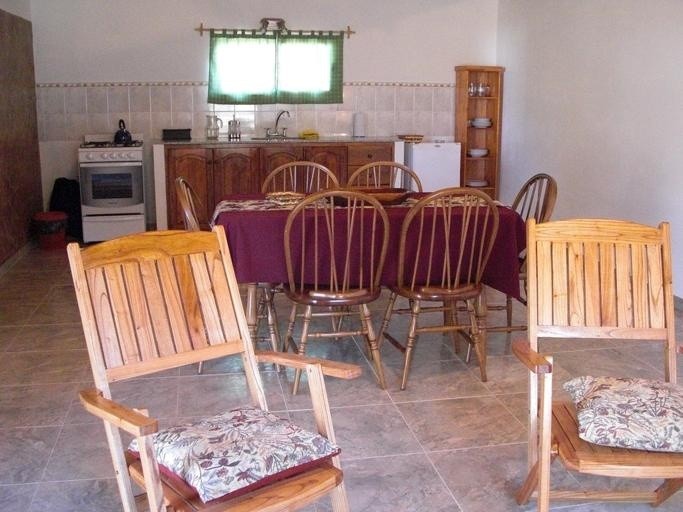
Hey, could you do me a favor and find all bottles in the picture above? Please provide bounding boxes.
[227,114,241,139]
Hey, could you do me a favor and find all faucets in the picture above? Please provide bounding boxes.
[274,110,290,136]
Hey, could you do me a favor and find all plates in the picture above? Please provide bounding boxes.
[466,147,488,158]
[470,118,492,129]
[464,180,490,187]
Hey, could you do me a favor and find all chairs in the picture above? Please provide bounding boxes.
[517,218,682,511]
[175,160,557,395]
[66,226,349,512]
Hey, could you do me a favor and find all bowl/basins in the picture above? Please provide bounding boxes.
[397,134,424,143]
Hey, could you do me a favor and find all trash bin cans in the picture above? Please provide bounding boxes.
[32,211,66,250]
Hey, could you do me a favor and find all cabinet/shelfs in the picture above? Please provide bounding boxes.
[164,142,394,230]
[455,66,506,200]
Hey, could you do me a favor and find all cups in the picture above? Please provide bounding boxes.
[468,81,491,98]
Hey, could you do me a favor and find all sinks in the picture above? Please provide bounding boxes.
[250,137,306,140]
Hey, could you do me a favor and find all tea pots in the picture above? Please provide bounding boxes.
[113,118,132,143]
[204,114,223,140]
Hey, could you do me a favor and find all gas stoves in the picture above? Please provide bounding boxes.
[80,133,144,148]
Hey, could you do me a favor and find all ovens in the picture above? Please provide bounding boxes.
[78,161,144,209]
